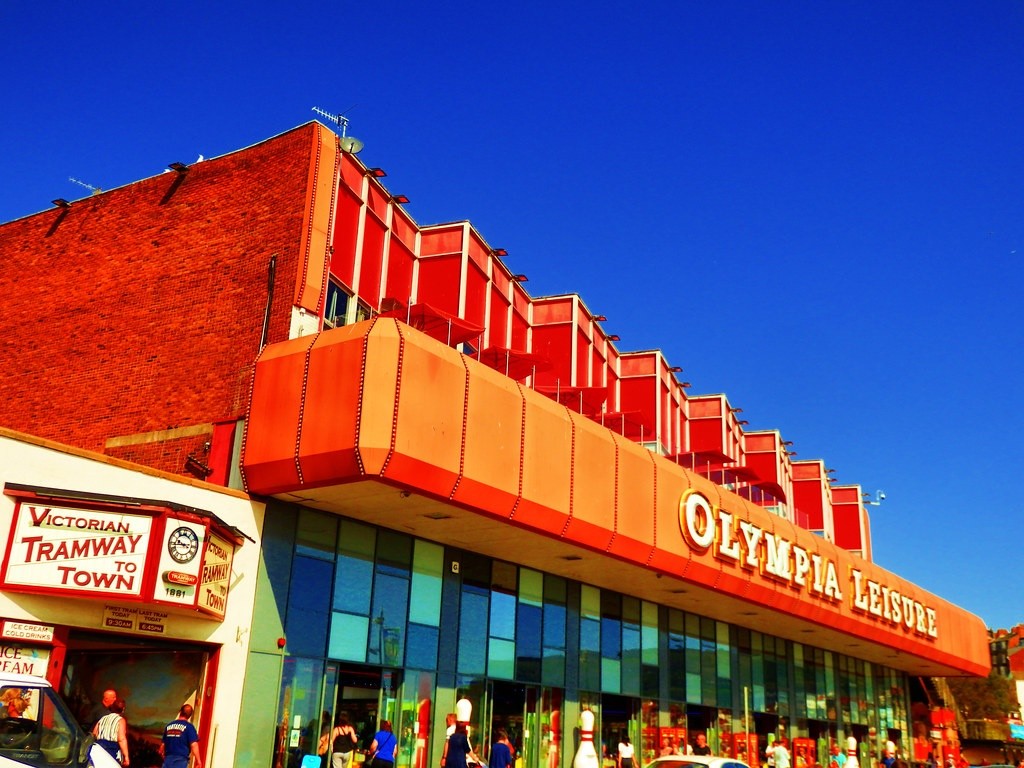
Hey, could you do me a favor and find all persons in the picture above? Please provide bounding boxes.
[88,695,130,768]
[1,686,32,720]
[162,704,202,768]
[832,747,846,768]
[602,733,639,768]
[296,709,331,768]
[879,749,908,768]
[924,751,955,768]
[766,739,791,768]
[367,720,398,768]
[796,746,807,766]
[659,734,712,757]
[330,712,358,768]
[440,714,516,768]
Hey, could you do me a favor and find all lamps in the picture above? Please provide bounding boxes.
[789,452,797,455]
[737,420,748,424]
[514,273,529,283]
[730,408,743,412]
[494,248,508,256]
[52,198,71,207]
[683,382,691,387]
[861,493,870,496]
[168,162,188,171]
[595,316,607,321]
[672,367,682,372]
[393,194,410,203]
[825,469,835,472]
[371,168,387,176]
[862,500,870,503]
[610,335,620,341]
[828,479,837,482]
[784,441,793,446]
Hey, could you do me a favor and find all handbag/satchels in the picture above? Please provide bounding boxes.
[366,731,392,766]
[340,726,358,749]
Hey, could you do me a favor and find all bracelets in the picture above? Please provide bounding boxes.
[442,756,447,759]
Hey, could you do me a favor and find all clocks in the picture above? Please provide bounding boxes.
[169,527,199,564]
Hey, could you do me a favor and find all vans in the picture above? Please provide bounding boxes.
[0,672,122,768]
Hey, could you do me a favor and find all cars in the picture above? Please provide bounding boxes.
[644,755,751,768]
